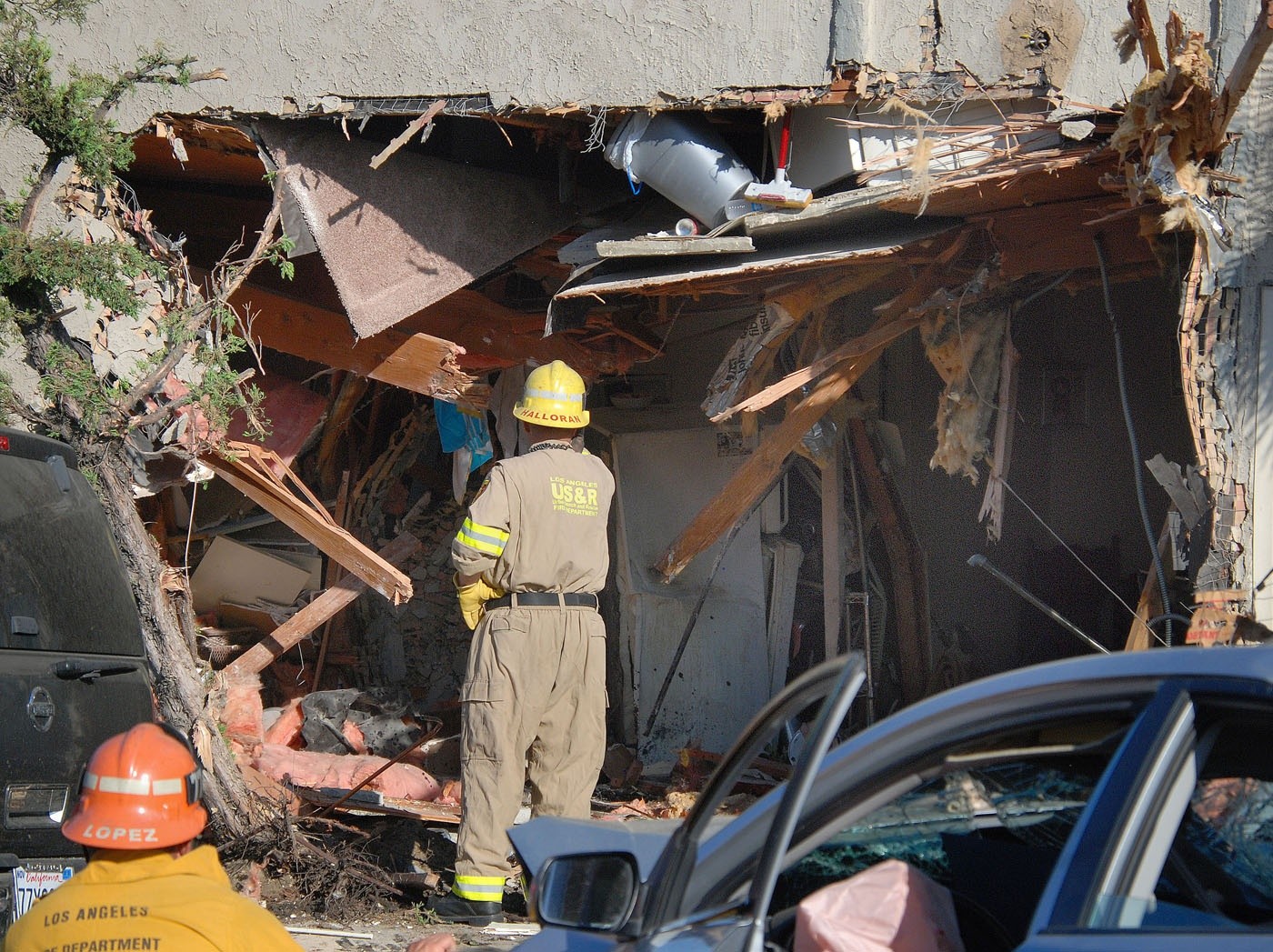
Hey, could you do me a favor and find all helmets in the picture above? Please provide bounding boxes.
[61,723,208,850]
[512,360,590,428]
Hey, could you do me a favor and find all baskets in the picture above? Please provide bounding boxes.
[609,392,652,409]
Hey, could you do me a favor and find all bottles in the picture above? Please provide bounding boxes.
[724,200,772,220]
[675,218,700,237]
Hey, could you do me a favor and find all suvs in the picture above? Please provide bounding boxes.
[0,425,166,951]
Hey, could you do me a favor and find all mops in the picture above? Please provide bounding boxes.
[744,107,813,209]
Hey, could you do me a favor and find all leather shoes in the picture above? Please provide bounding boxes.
[420,889,504,927]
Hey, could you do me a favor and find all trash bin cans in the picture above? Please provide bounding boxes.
[606,110,760,230]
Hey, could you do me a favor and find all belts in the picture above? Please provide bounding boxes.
[485,589,597,611]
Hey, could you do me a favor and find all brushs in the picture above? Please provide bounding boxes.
[744,106,813,209]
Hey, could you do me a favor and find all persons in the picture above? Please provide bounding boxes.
[4,722,457,952]
[426,360,615,926]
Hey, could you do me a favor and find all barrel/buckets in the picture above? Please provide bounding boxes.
[604,108,758,230]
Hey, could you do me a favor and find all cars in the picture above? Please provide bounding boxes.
[529,637,1272,950]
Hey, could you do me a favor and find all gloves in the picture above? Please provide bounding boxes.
[452,569,505,630]
